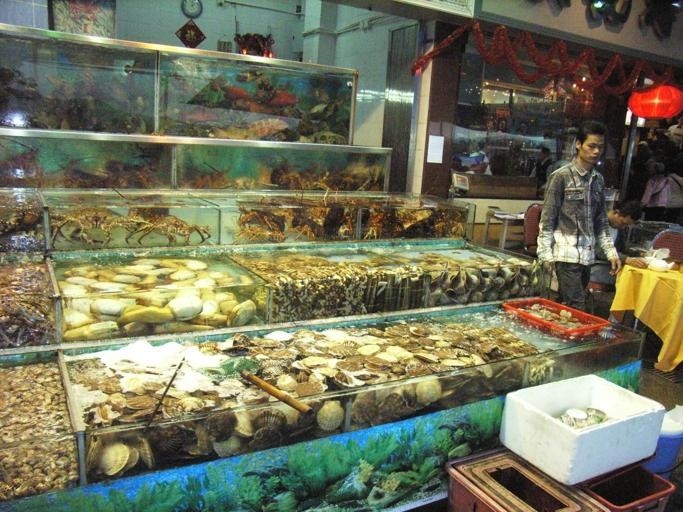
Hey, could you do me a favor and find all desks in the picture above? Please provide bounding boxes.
[620,257,682,371]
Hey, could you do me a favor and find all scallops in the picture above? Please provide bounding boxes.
[64,319,555,479]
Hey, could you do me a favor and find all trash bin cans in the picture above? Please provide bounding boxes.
[444,447,678,512]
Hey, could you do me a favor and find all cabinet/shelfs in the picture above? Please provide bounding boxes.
[482,209,522,254]
[0,22,647,512]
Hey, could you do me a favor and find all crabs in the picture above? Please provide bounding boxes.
[49,208,122,249]
[109,215,211,247]
[112,187,169,219]
[234,188,467,242]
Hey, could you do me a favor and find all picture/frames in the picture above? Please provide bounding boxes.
[46,0,117,39]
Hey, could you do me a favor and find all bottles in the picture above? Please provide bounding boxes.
[509,139,540,150]
[458,114,507,156]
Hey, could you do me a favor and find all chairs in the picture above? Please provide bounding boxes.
[522,201,540,257]
[649,226,682,267]
[584,281,617,317]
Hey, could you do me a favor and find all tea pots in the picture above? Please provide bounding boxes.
[645,258,675,272]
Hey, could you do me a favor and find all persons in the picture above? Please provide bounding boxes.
[588,200,649,323]
[624,116,683,227]
[470,138,493,176]
[529,148,553,195]
[536,121,622,312]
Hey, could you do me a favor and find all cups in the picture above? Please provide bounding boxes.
[551,406,614,431]
[645,256,655,262]
[536,128,544,137]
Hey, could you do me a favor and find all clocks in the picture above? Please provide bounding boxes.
[180,0,203,18]
